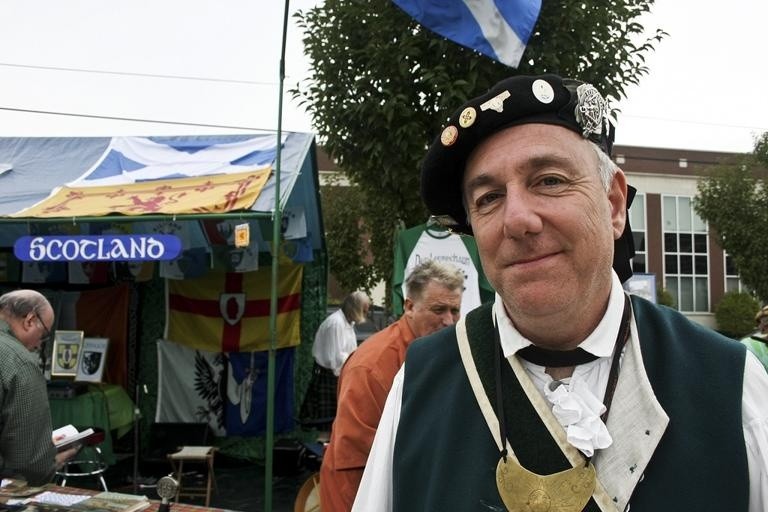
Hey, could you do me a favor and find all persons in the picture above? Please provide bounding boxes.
[740,305,768,375]
[0,289,78,487]
[320,260,462,512]
[352,74,768,512]
[299,292,370,439]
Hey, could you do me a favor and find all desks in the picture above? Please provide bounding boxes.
[47,380,138,488]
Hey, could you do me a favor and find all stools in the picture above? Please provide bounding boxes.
[53,427,107,491]
[165,445,222,507]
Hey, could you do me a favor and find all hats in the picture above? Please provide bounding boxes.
[420,74,615,219]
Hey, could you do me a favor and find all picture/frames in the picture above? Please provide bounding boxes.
[48,329,111,385]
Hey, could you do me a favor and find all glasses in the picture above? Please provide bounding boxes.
[23,312,51,343]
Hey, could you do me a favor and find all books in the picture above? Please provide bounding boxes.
[52,424,95,453]
[71,491,150,512]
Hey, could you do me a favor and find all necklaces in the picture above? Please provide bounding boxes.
[494,292,632,512]
